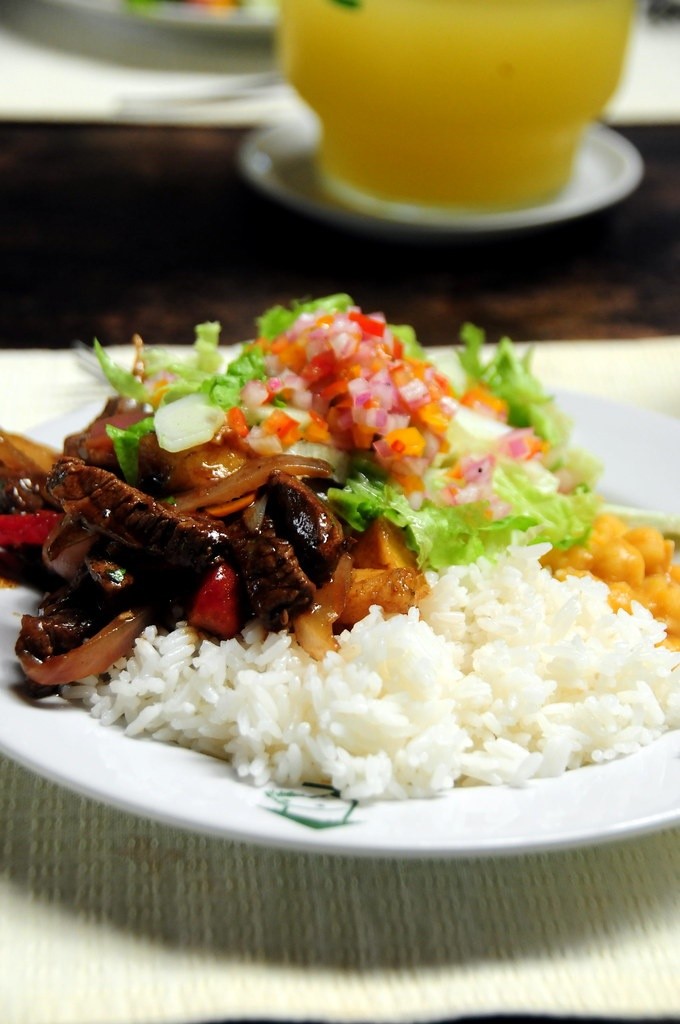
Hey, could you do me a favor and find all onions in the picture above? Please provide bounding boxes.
[0,430,352,687]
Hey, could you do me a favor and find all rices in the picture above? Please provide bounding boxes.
[59,540,680,803]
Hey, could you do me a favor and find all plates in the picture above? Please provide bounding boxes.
[236,114,645,242]
[0,388,680,860]
[47,0,277,42]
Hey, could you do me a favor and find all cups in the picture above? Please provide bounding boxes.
[270,0,634,212]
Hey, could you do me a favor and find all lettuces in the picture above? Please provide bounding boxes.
[89,290,600,573]
